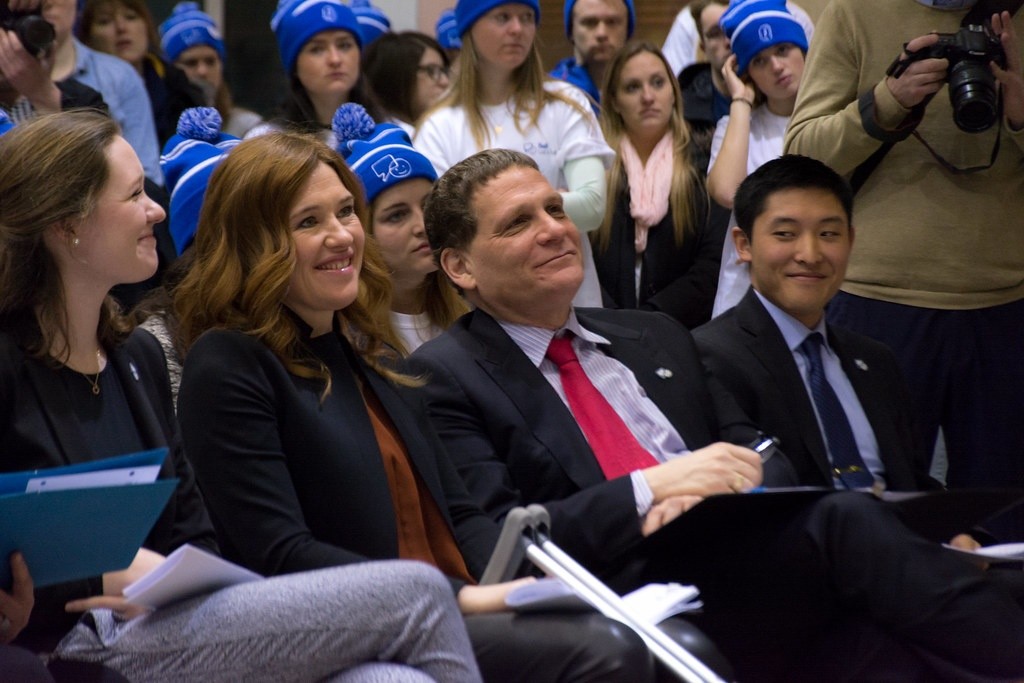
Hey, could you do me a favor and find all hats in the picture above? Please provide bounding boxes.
[343,0,392,55]
[158,2,224,62]
[332,100,439,209]
[270,0,364,77]
[566,0,634,43]
[549,57,599,113]
[455,0,541,41]
[720,0,810,77]
[158,105,246,255]
[434,10,463,48]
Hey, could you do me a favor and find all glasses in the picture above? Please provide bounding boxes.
[420,63,450,82]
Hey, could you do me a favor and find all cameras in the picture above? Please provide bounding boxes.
[925,23,1008,134]
[0,0,56,56]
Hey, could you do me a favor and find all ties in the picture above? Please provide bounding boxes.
[799,330,875,492]
[544,330,660,479]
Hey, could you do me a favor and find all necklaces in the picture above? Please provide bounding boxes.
[50,343,101,395]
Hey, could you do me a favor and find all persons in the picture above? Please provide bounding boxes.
[332,102,472,356]
[176,131,739,683]
[1,1,811,331]
[405,153,1024,683]
[135,106,243,418]
[691,155,993,546]
[783,0,1024,547]
[0,107,483,683]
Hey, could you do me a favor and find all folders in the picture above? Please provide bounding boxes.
[1,443,181,593]
[592,487,845,578]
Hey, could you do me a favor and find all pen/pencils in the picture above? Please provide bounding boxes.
[739,430,781,465]
